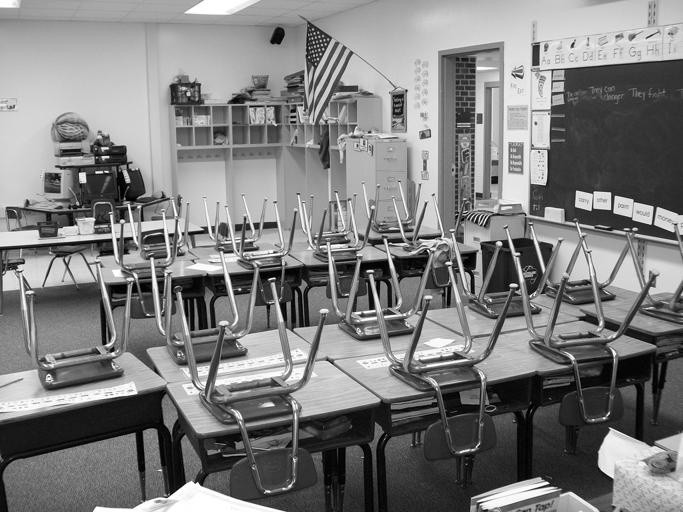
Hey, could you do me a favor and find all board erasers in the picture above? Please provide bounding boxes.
[594,224,614,231]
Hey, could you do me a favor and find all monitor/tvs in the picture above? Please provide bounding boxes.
[41,169,76,200]
[117,168,145,199]
[80,167,118,201]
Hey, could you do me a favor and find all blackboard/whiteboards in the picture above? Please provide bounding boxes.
[526,56,683,245]
[508,142,525,174]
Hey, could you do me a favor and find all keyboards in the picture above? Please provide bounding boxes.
[137,197,155,203]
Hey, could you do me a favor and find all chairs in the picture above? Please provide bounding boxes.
[2,179,681,362]
[364,261,520,463]
[173,276,333,497]
[12,258,135,391]
[511,248,661,427]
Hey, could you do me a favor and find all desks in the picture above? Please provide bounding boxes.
[577,291,681,425]
[166,362,383,512]
[471,320,659,483]
[2,351,169,512]
[333,339,536,512]
[144,328,326,388]
[613,433,681,508]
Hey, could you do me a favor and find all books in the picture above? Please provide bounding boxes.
[248,69,310,125]
[468,476,561,512]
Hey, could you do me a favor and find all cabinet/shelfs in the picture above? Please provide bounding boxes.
[345,135,408,229]
[168,96,383,151]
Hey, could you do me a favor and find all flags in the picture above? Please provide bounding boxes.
[305,22,354,126]
